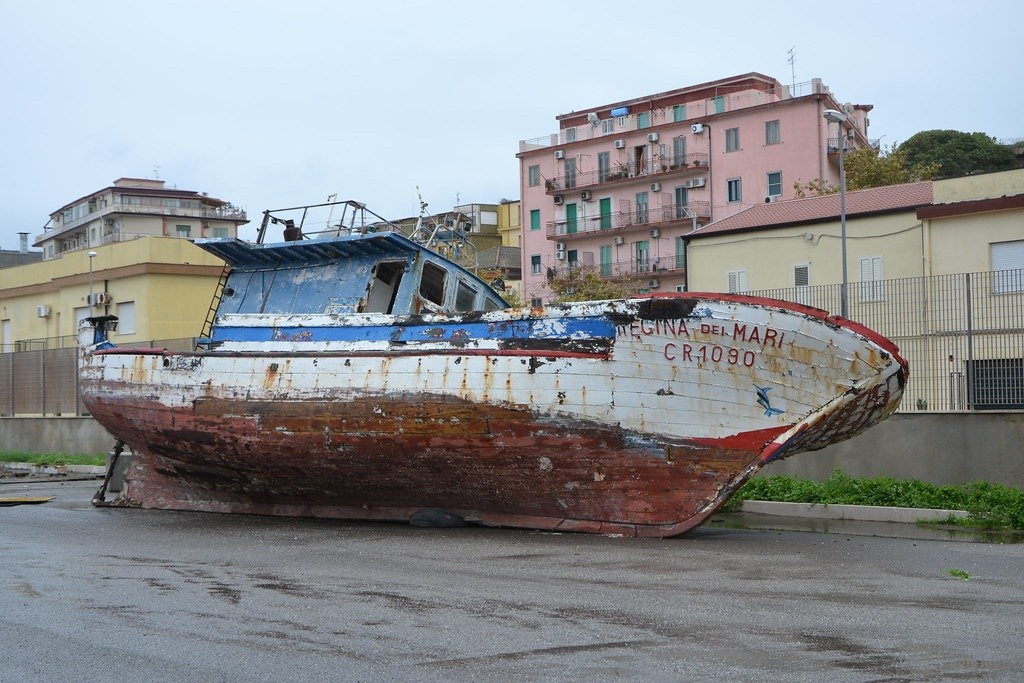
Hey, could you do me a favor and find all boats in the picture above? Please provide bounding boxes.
[76,201,907,540]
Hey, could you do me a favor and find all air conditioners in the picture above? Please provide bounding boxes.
[694,177,706,188]
[556,242,566,250]
[650,229,659,238]
[614,139,626,149]
[567,286,576,294]
[614,236,624,245]
[554,195,564,205]
[581,190,592,201]
[554,149,565,159]
[650,182,662,192]
[685,179,694,189]
[764,196,776,203]
[86,292,96,306]
[649,278,660,289]
[691,124,704,134]
[36,305,49,318]
[96,293,111,305]
[647,133,659,143]
[557,252,566,260]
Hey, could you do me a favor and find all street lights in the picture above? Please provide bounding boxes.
[824,108,852,320]
[88,250,98,316]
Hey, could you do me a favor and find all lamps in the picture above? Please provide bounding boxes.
[490,277,506,293]
